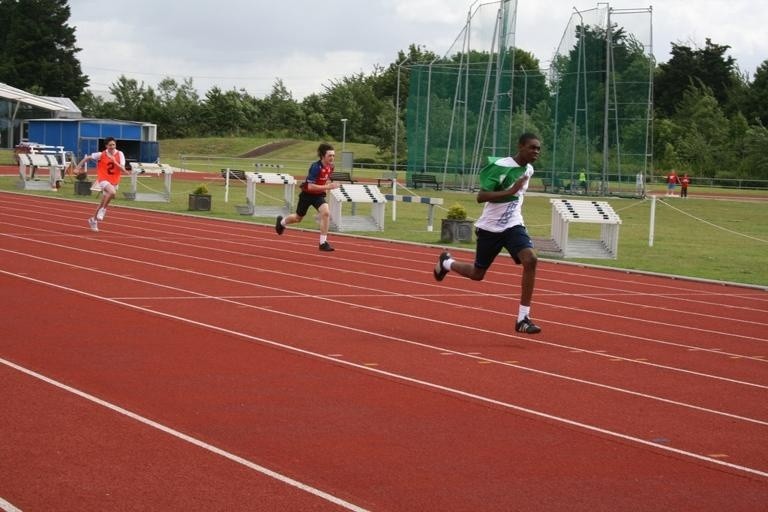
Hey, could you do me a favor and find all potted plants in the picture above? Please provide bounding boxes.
[441,203,475,242]
[74,171,92,195]
[189,184,212,212]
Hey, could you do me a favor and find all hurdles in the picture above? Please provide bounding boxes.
[16,142,66,192]
[124,162,173,202]
[533,199,622,262]
[314,184,443,233]
[221,168,298,217]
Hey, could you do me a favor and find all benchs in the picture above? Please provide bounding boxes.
[412,174,439,190]
[330,171,354,184]
[542,178,564,192]
[220,168,247,184]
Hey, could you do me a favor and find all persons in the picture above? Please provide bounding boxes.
[579,168,587,194]
[636,171,643,196]
[666,169,681,196]
[680,173,689,198]
[275,143,338,251]
[73,137,125,232]
[433,133,541,333]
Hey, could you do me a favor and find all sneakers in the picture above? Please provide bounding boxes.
[515,316,541,334]
[275,216,286,235]
[97,208,106,220]
[319,241,334,251]
[88,218,98,232]
[434,251,450,282]
